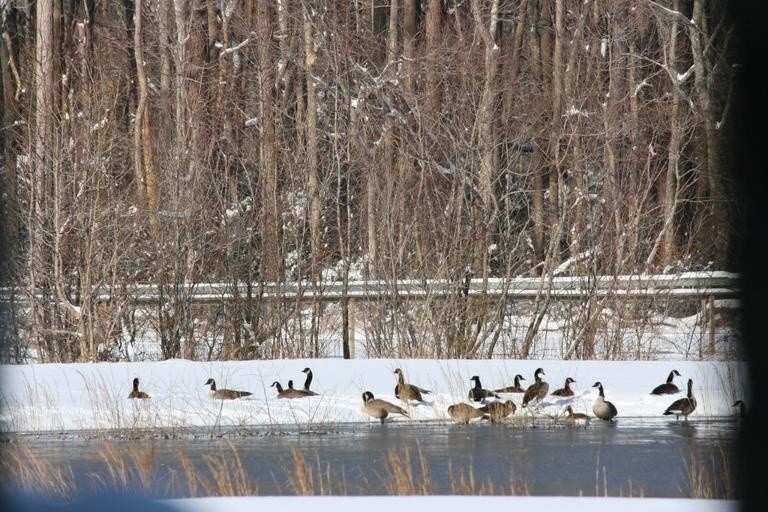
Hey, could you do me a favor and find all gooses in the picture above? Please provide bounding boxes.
[270,367,319,400]
[362,391,409,425]
[651,369,683,395]
[731,400,746,429]
[447,368,576,426]
[128,378,150,399]
[562,405,590,422]
[592,382,618,424]
[662,378,697,422]
[392,368,431,404]
[203,378,252,400]
